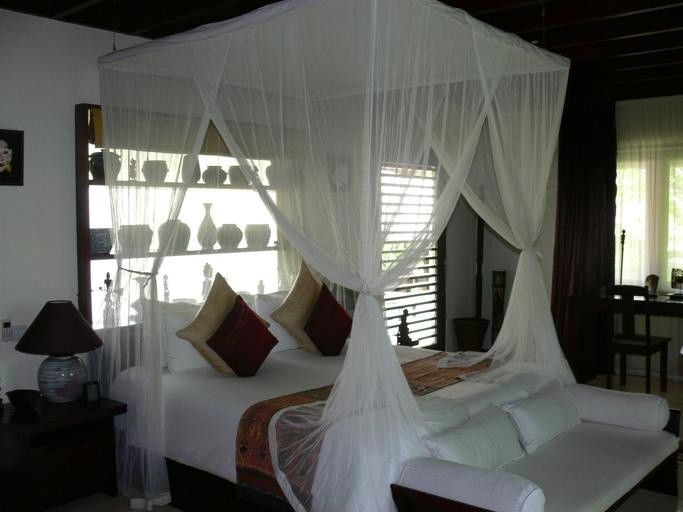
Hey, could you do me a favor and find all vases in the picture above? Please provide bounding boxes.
[88,147,280,254]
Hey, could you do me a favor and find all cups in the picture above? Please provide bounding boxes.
[645,276,659,293]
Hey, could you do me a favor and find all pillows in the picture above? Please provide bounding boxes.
[208,295,277,377]
[134,291,255,378]
[306,283,350,355]
[176,272,270,375]
[422,406,523,469]
[271,257,323,355]
[506,378,580,457]
[250,291,299,352]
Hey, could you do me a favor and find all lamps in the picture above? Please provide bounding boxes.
[14,297,103,403]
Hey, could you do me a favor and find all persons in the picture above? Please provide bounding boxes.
[0,138,13,174]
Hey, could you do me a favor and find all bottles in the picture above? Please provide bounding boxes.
[279,267,297,292]
[196,203,219,251]
[255,280,264,295]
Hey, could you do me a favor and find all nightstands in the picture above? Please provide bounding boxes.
[0,391,128,512]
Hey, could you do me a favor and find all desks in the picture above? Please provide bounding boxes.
[572,285,683,383]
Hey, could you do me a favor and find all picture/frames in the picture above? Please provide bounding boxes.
[0,125,27,190]
[83,380,101,405]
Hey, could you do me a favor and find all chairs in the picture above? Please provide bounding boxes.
[604,283,667,394]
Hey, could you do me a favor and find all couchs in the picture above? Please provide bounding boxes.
[393,383,683,512]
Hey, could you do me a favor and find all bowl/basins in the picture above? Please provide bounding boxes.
[6,389,45,409]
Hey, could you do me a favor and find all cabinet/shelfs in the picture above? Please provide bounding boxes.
[74,102,309,332]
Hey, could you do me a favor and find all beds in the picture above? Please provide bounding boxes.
[99,8,575,512]
